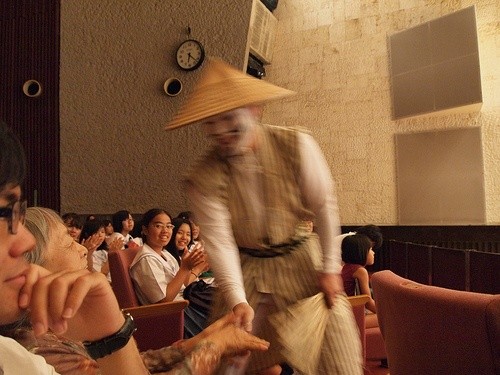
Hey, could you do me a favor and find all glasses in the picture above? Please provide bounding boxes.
[0,201,27,233]
[154,223,175,230]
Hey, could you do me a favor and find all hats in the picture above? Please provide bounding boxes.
[166,59,297,131]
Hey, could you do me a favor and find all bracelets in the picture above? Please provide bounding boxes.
[191,272,198,278]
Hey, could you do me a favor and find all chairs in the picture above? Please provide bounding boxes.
[107,248,500,375]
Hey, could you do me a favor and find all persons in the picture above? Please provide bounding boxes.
[162,57,365,375]
[336,224,382,330]
[0,198,270,375]
[0,117,152,375]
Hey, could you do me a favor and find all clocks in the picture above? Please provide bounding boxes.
[176,39,205,71]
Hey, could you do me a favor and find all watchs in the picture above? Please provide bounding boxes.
[82,308,136,360]
